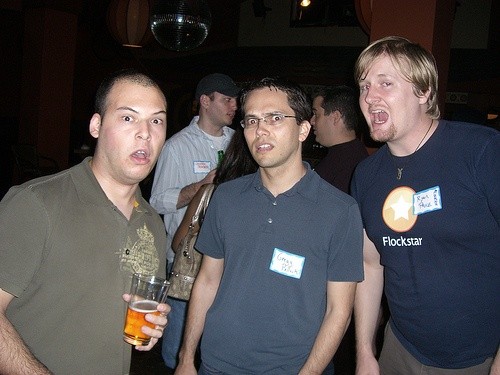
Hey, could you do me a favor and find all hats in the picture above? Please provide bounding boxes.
[196,74,243,97]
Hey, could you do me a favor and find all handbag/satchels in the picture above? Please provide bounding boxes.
[167,183,215,300]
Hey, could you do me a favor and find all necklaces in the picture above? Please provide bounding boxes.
[397,120,434,180]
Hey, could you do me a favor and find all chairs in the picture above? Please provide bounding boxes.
[11,143,60,184]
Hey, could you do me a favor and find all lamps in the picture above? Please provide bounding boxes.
[106,0,156,49]
[150,0,213,53]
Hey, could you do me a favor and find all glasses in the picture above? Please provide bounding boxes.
[240,114,297,128]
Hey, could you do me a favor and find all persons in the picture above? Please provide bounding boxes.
[171,126,258,251]
[354,36,500,375]
[149,73,243,375]
[311,85,390,375]
[0,73,171,375]
[174,76,364,375]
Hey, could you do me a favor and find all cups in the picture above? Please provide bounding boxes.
[122,273,172,346]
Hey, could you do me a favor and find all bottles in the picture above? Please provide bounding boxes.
[217,150,225,168]
